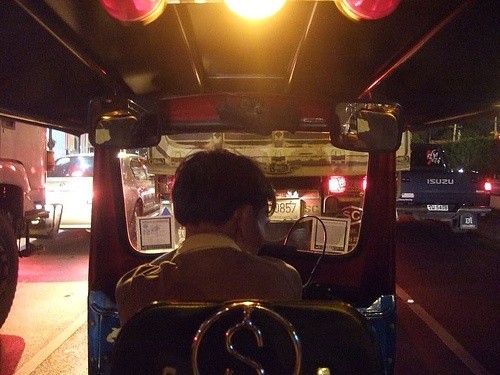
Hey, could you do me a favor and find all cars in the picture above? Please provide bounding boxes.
[36,151,161,238]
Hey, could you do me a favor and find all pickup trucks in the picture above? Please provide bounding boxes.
[396,142,491,234]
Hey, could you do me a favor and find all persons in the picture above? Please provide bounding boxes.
[116,148,304,321]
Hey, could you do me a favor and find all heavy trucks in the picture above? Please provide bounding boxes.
[153,110,413,249]
[1,114,56,330]
